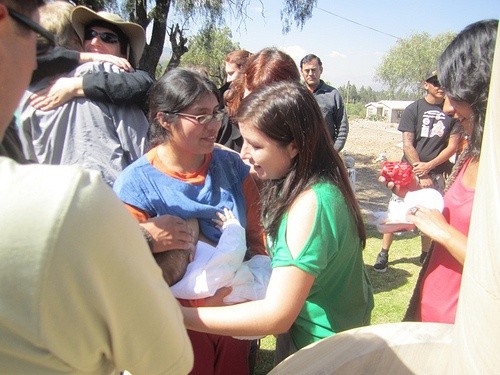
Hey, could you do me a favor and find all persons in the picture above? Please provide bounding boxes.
[150,208,273,304]
[108,68,270,374]
[15,1,150,188]
[248,47,298,92]
[299,55,348,155]
[28,6,155,111]
[372,72,465,271]
[400,19,500,326]
[215,49,251,153]
[182,81,377,350]
[0,0,193,374]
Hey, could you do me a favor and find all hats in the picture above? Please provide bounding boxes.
[425,66,437,79]
[69,6,146,68]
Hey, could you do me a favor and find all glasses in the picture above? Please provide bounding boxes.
[84,30,123,44]
[161,108,225,123]
[3,8,57,56]
[427,80,440,87]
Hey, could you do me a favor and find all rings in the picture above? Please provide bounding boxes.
[410,203,418,218]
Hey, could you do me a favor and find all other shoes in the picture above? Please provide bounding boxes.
[419,255,425,265]
[373,252,389,272]
